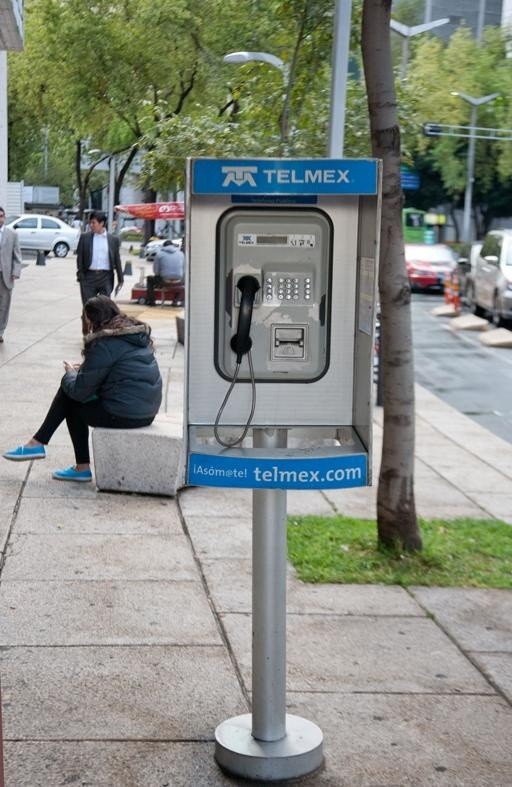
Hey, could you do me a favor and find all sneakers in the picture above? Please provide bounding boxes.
[53,466,92,483]
[3,446,45,462]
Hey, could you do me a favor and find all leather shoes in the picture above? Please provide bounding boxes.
[0,336,3,342]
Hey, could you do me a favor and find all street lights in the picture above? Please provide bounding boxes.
[222,49,293,101]
[449,91,502,244]
[388,16,450,85]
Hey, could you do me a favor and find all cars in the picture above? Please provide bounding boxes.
[401,208,512,328]
[0,214,80,258]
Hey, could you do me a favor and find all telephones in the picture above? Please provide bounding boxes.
[231,222,323,378]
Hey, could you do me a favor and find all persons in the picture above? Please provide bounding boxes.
[2,295,163,481]
[144,239,185,305]
[75,210,125,339]
[0,206,24,344]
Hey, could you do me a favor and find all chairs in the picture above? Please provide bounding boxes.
[162,275,185,308]
